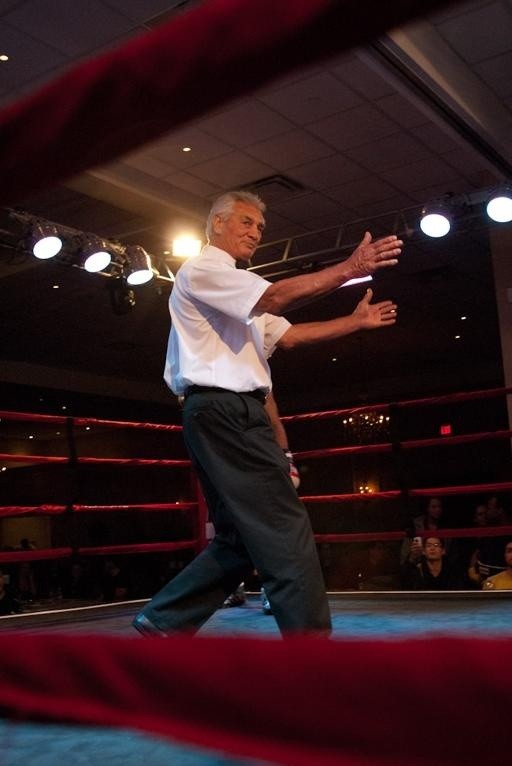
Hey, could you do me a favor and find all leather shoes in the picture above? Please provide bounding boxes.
[131,612,169,639]
[218,586,273,616]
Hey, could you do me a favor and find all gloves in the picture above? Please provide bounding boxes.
[283,449,299,489]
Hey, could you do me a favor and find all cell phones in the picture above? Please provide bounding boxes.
[413,537,422,544]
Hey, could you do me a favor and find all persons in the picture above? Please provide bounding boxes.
[262,359,301,492]
[0,485,511,613]
[131,186,403,637]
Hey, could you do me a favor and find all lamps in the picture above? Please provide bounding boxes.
[78,235,112,273]
[123,244,154,286]
[109,279,136,316]
[419,202,452,238]
[486,187,512,223]
[28,219,64,260]
